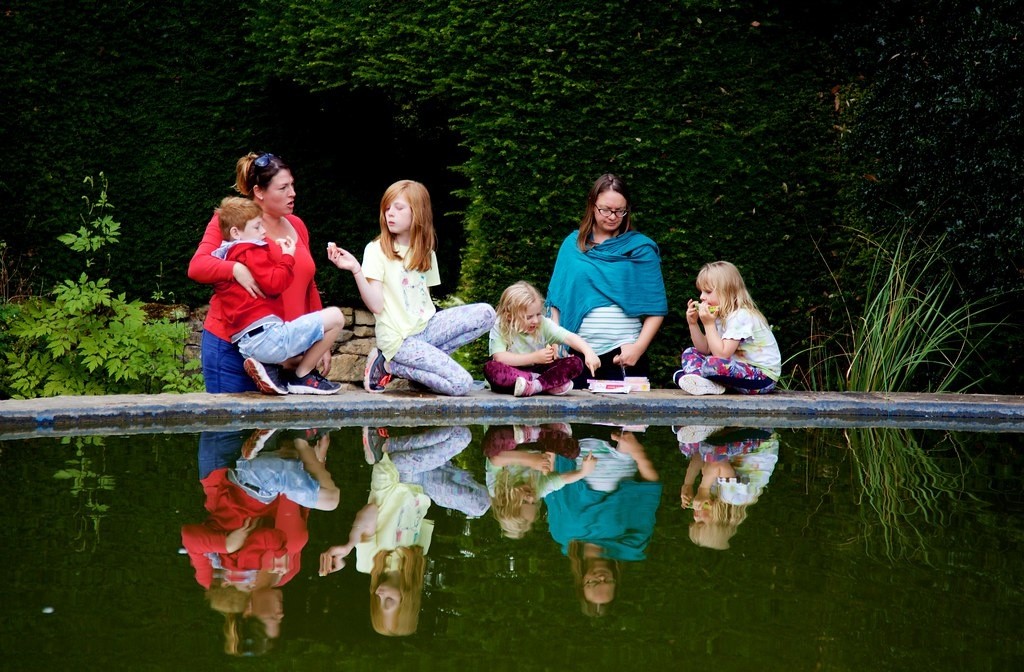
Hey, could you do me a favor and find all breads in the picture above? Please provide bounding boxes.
[328,242,338,257]
[319,555,329,577]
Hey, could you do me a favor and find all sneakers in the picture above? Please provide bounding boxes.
[549,422,573,439]
[241,428,284,460]
[363,426,389,466]
[513,377,536,397]
[243,357,289,395]
[364,347,391,393]
[514,425,540,445]
[548,380,573,396]
[287,366,342,395]
[408,379,432,393]
[287,427,342,447]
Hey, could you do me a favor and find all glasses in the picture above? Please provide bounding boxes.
[252,153,275,188]
[583,576,617,587]
[594,203,627,218]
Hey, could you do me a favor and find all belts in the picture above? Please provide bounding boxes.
[240,480,260,494]
[248,325,264,338]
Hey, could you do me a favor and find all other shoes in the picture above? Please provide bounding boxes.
[673,370,685,387]
[678,374,726,395]
[672,425,682,434]
[677,426,726,444]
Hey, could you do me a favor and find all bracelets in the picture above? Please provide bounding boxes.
[353,268,361,275]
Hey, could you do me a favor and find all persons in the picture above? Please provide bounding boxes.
[210,196,343,396]
[672,425,779,550]
[483,281,601,396]
[180,429,340,658]
[482,423,598,539]
[543,424,663,617]
[327,181,496,396]
[187,154,332,394]
[544,175,668,391]
[672,261,782,396]
[320,425,491,636]
